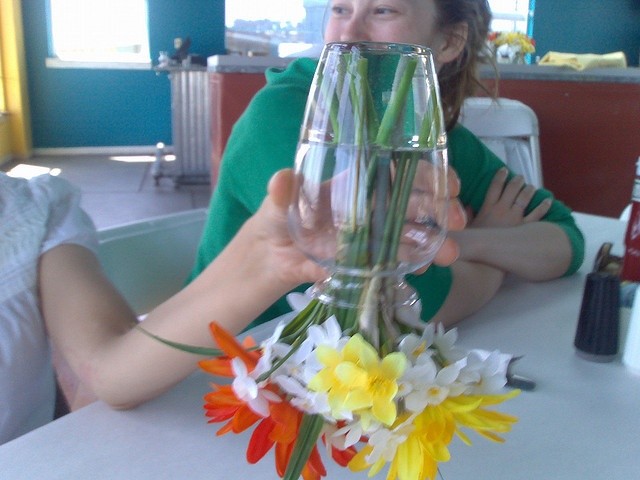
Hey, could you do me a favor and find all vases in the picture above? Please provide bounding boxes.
[285,40,450,313]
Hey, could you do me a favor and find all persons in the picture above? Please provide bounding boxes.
[186,0,587,342]
[0,154,469,451]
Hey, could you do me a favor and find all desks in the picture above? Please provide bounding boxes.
[0,209,640,478]
[203,53,639,221]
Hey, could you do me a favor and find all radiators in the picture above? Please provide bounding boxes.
[152,62,211,186]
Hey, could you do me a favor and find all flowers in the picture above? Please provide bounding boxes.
[133,52,526,480]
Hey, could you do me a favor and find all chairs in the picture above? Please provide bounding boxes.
[94,208,204,319]
[457,95,544,195]
